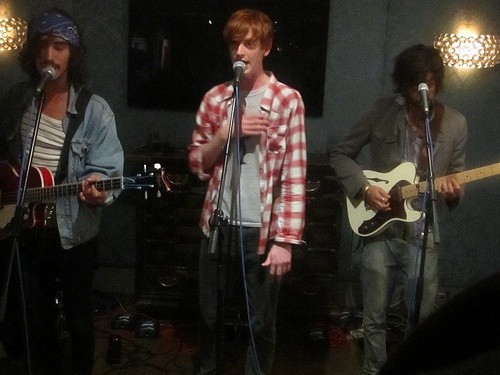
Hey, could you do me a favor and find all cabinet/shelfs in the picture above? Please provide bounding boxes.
[136,159,344,350]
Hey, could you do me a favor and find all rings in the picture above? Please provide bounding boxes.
[382,197,384,202]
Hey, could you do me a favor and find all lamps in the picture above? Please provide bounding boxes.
[0,17,27,53]
[432,32,500,68]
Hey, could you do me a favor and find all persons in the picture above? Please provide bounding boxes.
[187,9,307,375]
[0,11,124,375]
[330,45,468,375]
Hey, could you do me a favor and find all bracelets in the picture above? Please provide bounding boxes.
[361,185,370,200]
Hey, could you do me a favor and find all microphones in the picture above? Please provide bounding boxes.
[34,66,56,96]
[418,83,430,118]
[233,60,246,86]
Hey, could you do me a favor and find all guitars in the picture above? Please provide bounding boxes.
[346,161,500,238]
[0,159,171,240]
